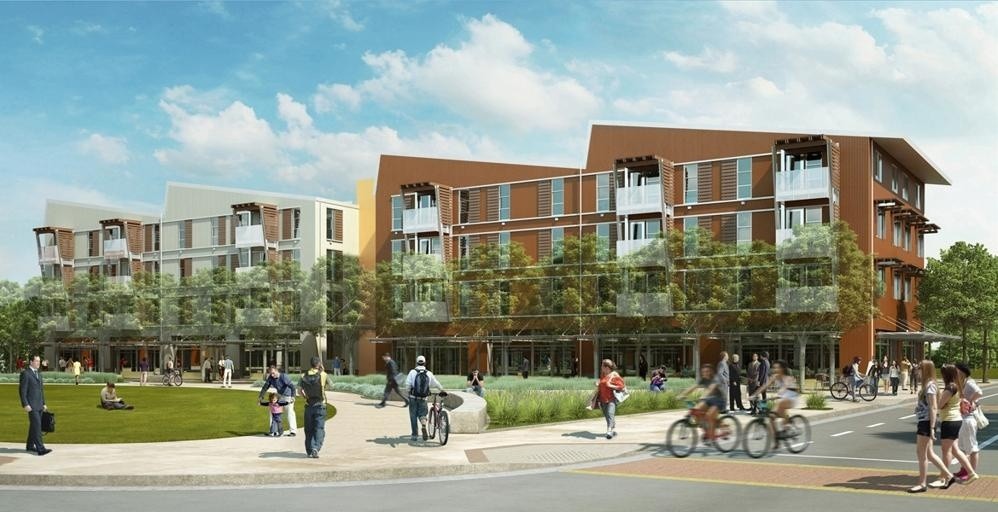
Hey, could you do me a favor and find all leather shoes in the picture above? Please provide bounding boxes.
[25,446,38,453]
[907,482,927,494]
[37,447,52,457]
[939,477,956,490]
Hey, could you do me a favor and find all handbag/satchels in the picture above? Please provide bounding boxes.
[613,384,631,406]
[393,371,407,387]
[959,398,973,416]
[39,409,56,434]
[973,405,990,431]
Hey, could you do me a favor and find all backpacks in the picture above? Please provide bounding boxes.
[841,362,856,378]
[302,368,325,403]
[409,367,431,398]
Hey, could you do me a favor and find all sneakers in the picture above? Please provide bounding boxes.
[410,435,418,441]
[961,474,980,486]
[952,467,978,481]
[263,429,297,438]
[421,424,428,441]
[927,478,946,489]
[607,431,617,440]
[308,448,319,459]
[375,400,411,409]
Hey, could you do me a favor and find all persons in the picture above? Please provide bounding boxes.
[201,355,234,388]
[466,367,485,398]
[297,356,333,458]
[100,383,133,410]
[594,359,624,440]
[16,355,93,386]
[375,352,409,407]
[139,357,149,387]
[332,355,341,376]
[19,353,52,456]
[848,354,983,493]
[406,355,445,441]
[257,365,297,436]
[166,356,174,386]
[638,354,681,394]
[676,350,799,448]
[521,355,529,379]
[259,392,293,437]
[119,358,128,371]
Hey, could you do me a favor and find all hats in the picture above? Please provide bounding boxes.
[416,355,426,364]
[955,362,973,378]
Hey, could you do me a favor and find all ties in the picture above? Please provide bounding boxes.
[33,369,39,378]
